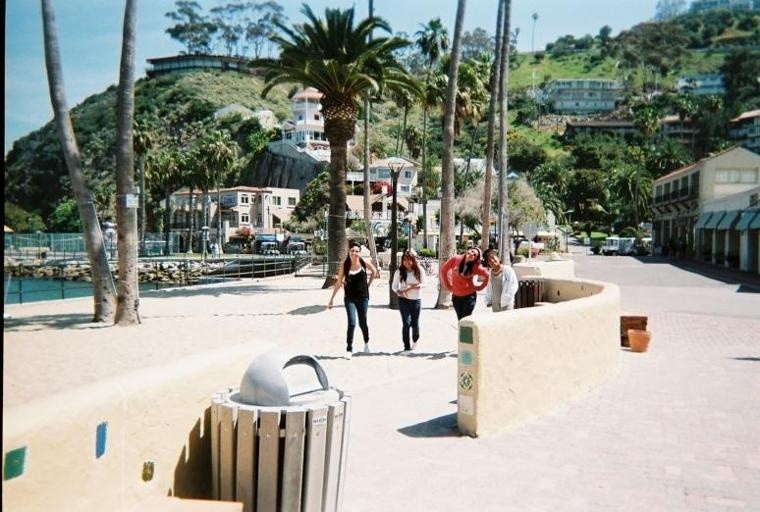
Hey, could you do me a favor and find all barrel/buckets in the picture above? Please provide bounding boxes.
[627,328,652,352]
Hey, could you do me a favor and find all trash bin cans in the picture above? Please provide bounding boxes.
[210,348,353,512]
[516,280,544,308]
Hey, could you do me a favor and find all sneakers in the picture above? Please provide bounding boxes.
[412,342,419,350]
[344,351,352,359]
[365,343,369,354]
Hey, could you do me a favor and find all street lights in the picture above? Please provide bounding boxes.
[568,210,573,226]
[387,157,405,309]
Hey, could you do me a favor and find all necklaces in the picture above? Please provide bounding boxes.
[492,268,501,276]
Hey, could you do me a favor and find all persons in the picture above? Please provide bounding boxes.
[390,248,423,356]
[441,245,488,321]
[328,241,377,358]
[482,248,520,313]
[210,241,218,258]
